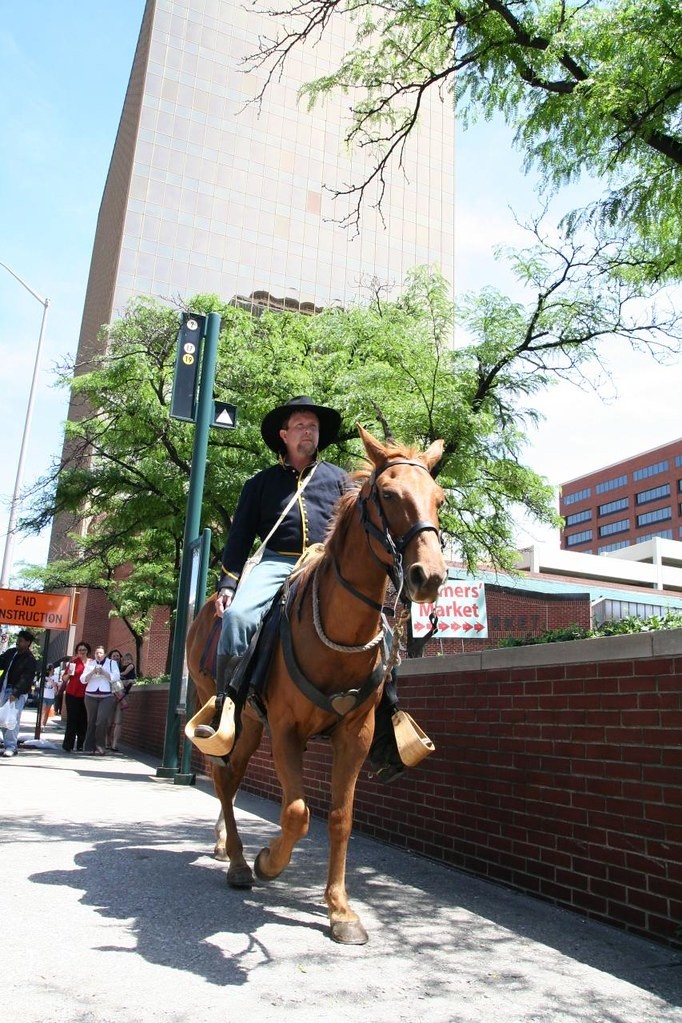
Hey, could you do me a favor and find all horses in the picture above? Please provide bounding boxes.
[186,421,449,945]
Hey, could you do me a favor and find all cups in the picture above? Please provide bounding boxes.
[68,662,76,676]
[95,665,102,678]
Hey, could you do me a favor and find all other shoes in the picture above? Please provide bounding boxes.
[375,738,432,783]
[117,691,125,702]
[3,749,18,757]
[41,725,46,733]
[62,744,72,752]
[107,741,119,751]
[77,746,83,751]
[120,703,128,710]
[95,746,105,754]
[194,724,230,767]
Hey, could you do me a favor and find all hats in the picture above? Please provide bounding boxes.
[261,395,342,455]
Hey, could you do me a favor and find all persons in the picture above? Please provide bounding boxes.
[62,642,93,753]
[80,645,120,756]
[196,396,406,783]
[0,631,37,757]
[40,664,58,733]
[106,649,135,751]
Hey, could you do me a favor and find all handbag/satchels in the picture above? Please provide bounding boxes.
[0,698,18,730]
[233,558,261,606]
[110,659,125,694]
